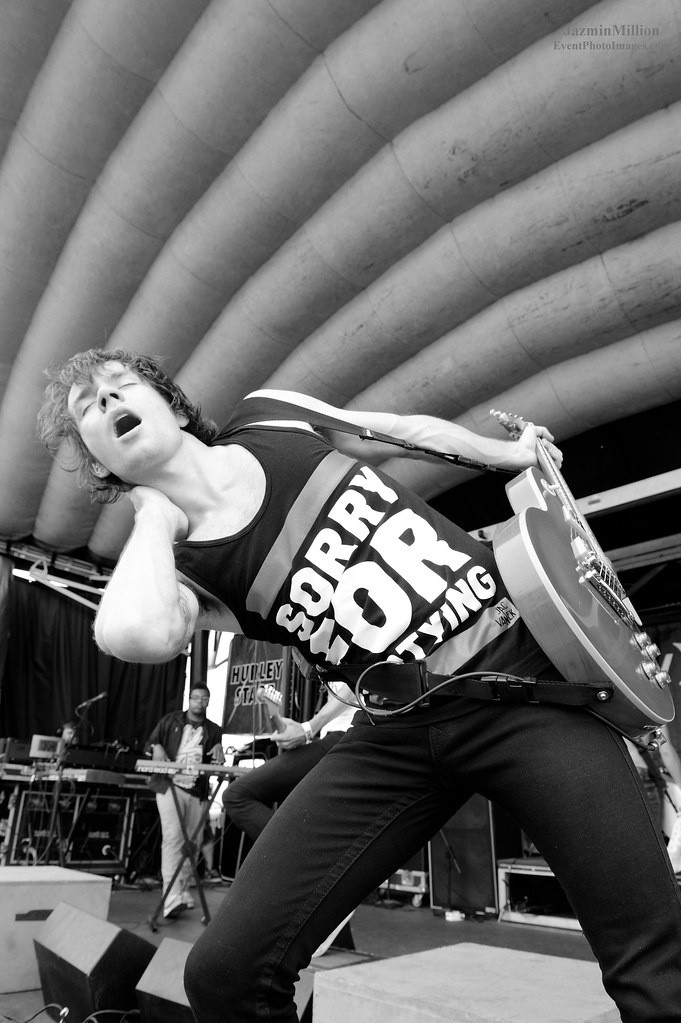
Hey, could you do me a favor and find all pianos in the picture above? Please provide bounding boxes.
[134,758,254,779]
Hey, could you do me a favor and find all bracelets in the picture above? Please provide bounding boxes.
[301,720,314,741]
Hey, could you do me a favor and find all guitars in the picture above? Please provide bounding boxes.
[485,405,679,742]
[258,683,286,734]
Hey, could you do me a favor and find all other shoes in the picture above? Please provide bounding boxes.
[181,891,195,908]
[163,899,187,918]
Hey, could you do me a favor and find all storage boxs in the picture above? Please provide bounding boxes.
[376,843,428,907]
[9,791,130,866]
[0,863,113,994]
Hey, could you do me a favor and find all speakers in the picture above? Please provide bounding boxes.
[31,902,316,1023]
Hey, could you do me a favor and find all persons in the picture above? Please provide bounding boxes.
[144,682,216,918]
[34,349,681,1023]
[42,722,91,861]
[623,724,681,833]
[222,681,366,837]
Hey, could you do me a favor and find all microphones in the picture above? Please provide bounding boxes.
[78,692,107,708]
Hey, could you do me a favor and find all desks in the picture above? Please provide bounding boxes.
[136,760,256,933]
